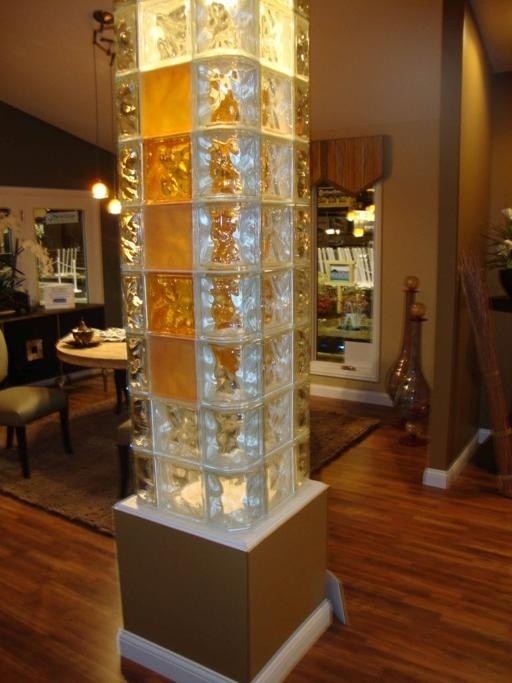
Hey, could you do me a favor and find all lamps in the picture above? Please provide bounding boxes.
[106,66,122,215]
[90,43,110,201]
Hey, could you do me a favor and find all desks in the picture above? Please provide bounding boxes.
[54,327,130,416]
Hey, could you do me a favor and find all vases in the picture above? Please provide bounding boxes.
[497,268,512,297]
[384,275,421,406]
[395,301,432,440]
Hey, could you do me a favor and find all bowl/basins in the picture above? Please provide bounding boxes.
[71,327,94,344]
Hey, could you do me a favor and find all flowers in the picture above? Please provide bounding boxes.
[474,206,512,271]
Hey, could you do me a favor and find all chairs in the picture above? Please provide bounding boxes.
[0,328,73,479]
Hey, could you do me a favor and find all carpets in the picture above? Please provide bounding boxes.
[0,395,381,541]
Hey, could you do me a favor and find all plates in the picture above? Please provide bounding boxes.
[61,336,101,347]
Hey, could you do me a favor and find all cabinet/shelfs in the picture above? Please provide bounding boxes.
[0,300,105,385]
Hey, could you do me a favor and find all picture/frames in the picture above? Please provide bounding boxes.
[325,259,357,287]
